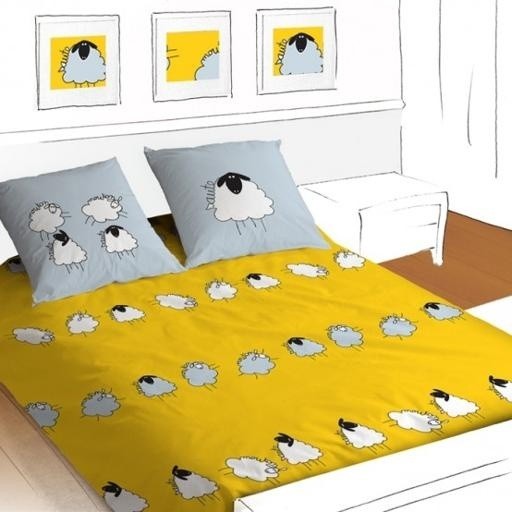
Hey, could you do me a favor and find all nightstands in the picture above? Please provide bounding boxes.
[301,169,451,269]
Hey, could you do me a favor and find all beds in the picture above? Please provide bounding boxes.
[0,213,512,512]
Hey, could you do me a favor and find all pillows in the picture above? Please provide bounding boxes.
[0,136,332,305]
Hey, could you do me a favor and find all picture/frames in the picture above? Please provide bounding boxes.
[151,11,233,103]
[254,6,340,96]
[33,13,122,110]
